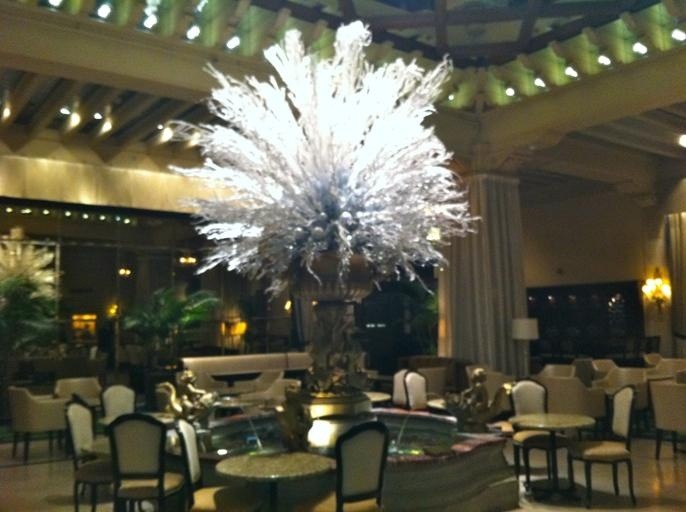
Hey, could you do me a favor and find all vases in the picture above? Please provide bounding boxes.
[286,243,382,422]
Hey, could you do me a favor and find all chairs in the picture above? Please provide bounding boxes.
[6,351,685,512]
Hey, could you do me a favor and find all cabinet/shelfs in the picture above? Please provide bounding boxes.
[526,278,646,375]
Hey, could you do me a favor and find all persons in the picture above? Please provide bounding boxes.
[79,323,91,337]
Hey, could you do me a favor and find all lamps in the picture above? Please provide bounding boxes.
[641,265,674,317]
[510,316,541,379]
